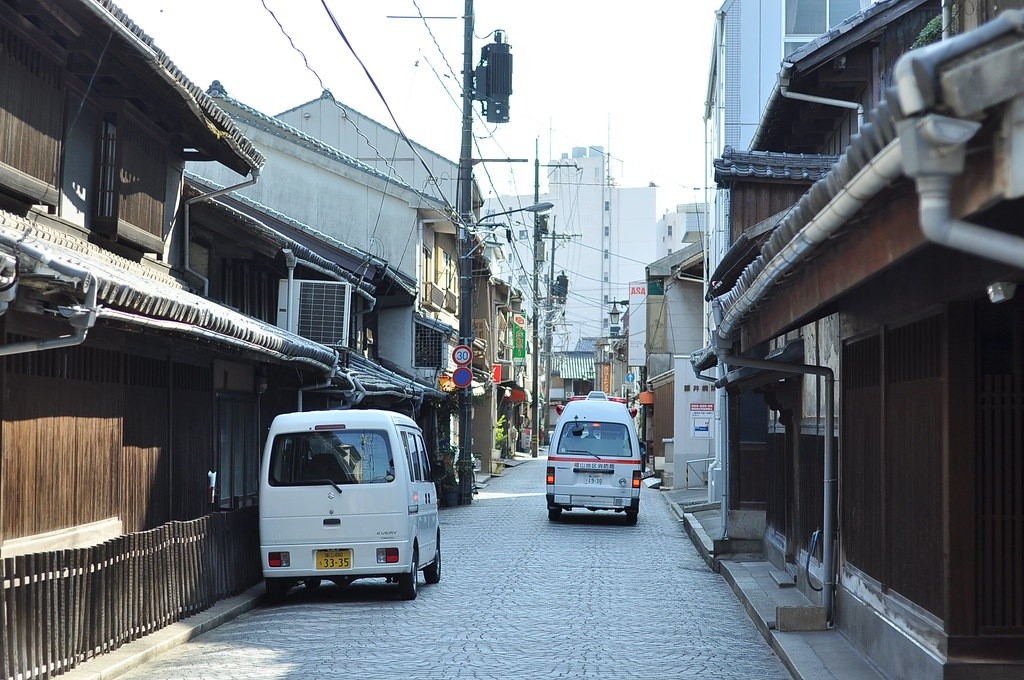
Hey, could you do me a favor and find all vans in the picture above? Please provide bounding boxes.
[258,408,447,602]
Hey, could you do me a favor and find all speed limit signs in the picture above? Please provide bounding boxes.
[453,345,473,367]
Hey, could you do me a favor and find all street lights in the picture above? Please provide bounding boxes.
[456,202,554,507]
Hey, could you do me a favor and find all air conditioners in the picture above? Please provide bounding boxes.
[275,277,354,348]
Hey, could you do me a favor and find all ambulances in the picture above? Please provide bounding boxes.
[544,390,643,525]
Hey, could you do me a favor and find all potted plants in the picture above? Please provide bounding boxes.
[490,415,507,460]
